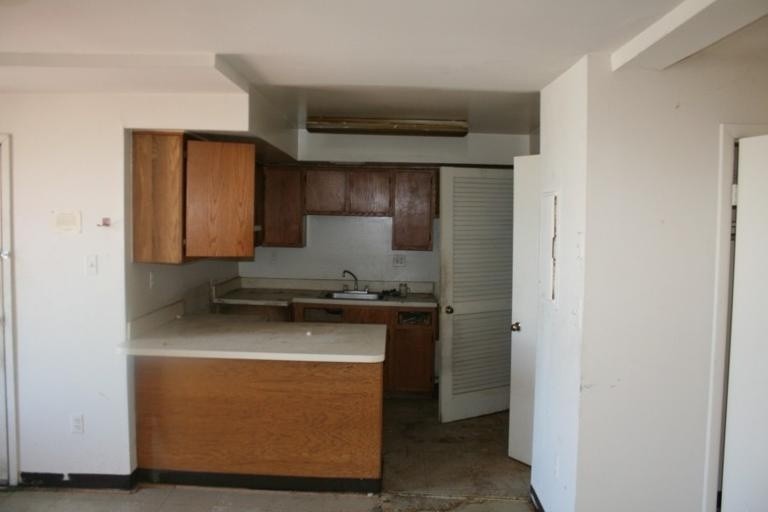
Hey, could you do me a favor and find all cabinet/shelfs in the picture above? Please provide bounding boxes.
[302,160,394,216]
[260,145,306,247]
[391,162,440,252]
[131,130,263,264]
[213,304,292,322]
[292,302,437,399]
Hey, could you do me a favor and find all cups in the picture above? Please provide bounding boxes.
[399,283,410,297]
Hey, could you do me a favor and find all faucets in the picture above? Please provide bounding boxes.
[341,268,368,294]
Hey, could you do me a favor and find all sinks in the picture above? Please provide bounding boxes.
[331,293,380,301]
